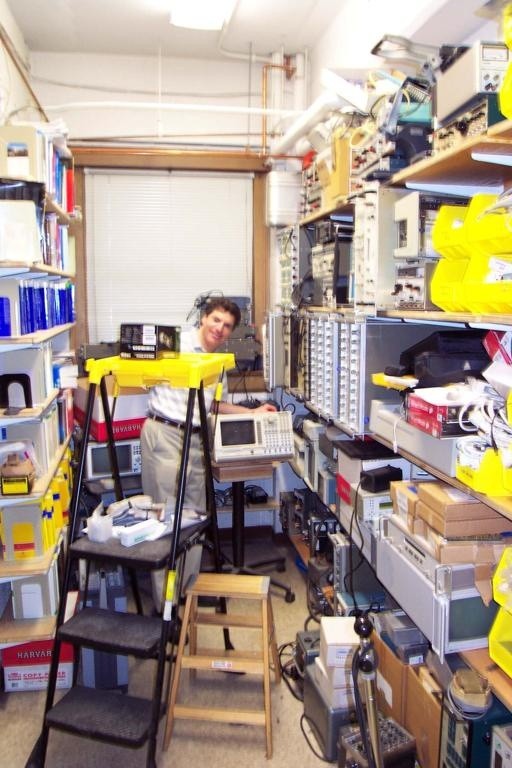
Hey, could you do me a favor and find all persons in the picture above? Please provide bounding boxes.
[140,296,278,646]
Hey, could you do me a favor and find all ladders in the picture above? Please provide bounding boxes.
[21,353,245,768]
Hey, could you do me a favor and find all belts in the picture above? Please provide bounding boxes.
[148,412,201,434]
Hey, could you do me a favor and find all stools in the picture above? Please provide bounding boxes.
[161,571,288,761]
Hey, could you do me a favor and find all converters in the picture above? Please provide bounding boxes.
[359,463,403,492]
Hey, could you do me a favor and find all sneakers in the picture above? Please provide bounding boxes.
[182,589,223,607]
[153,609,189,645]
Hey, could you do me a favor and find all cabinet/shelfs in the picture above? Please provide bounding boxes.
[0,119,86,694]
[270,10,511,768]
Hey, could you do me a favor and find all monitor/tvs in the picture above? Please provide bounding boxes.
[85,438,145,483]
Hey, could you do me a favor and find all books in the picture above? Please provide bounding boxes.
[0,118,76,480]
